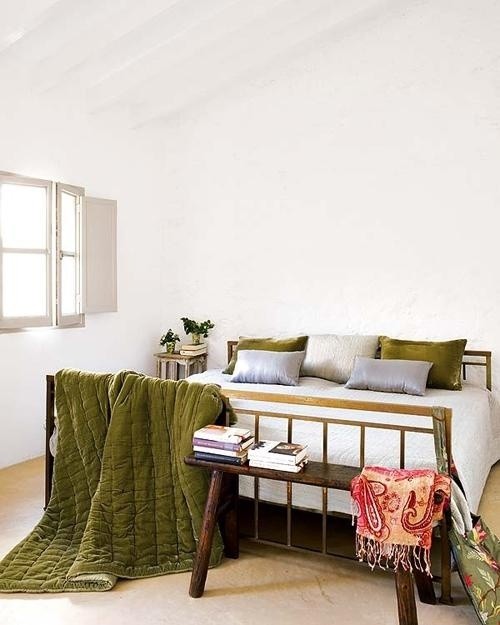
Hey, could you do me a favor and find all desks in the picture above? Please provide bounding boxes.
[154,350,207,380]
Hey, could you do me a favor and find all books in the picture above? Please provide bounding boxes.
[180,349,209,356]
[182,344,207,350]
[192,424,310,474]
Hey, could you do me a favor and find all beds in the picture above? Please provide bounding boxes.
[44,329,498,605]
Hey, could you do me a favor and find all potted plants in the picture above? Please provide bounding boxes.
[159,328,181,354]
[180,315,215,344]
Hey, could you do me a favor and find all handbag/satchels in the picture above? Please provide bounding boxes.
[447,511,499,625]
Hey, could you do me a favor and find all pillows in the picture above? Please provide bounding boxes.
[299,332,380,381]
[342,354,435,397]
[227,349,307,387]
[222,335,309,378]
[380,335,469,391]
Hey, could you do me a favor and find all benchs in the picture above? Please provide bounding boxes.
[180,447,453,625]
